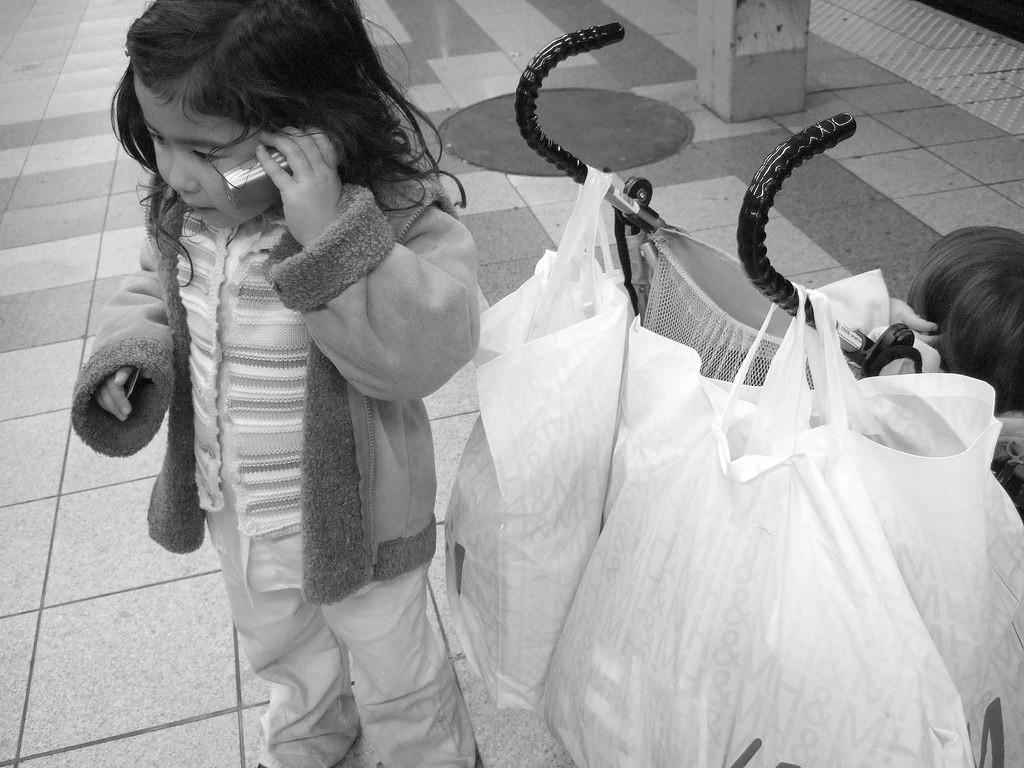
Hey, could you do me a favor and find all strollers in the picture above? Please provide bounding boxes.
[515,23,1023,516]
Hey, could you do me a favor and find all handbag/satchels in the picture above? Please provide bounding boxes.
[442,165,1024,768]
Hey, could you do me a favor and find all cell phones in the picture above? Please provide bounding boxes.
[222,148,294,210]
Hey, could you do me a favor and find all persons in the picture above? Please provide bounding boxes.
[71,0,481,768]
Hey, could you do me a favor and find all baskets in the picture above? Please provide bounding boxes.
[642,227,862,393]
